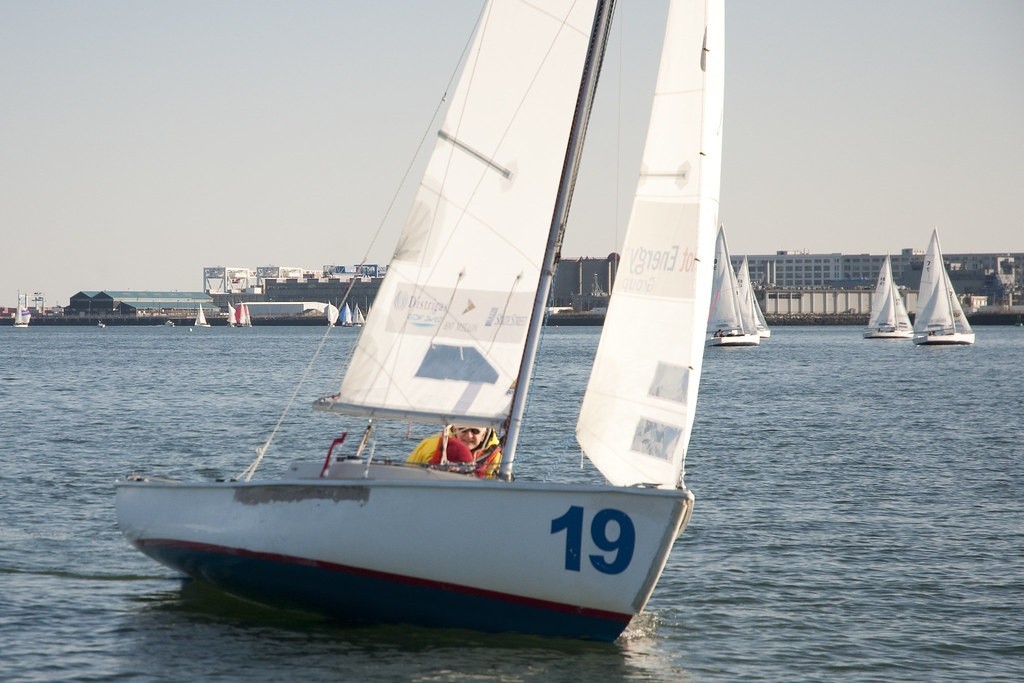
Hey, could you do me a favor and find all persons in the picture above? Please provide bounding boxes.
[405,425,502,479]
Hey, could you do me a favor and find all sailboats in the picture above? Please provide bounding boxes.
[705,222,770,346]
[324,300,340,327]
[352,303,365,327]
[13,298,31,327]
[195,303,211,327]
[863,251,915,337]
[226,300,252,328]
[912,226,976,344]
[114,0,725,644]
[339,302,353,327]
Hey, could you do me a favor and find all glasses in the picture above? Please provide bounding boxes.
[459,428,480,434]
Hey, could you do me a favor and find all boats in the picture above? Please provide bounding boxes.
[97,320,105,328]
[165,320,175,327]
